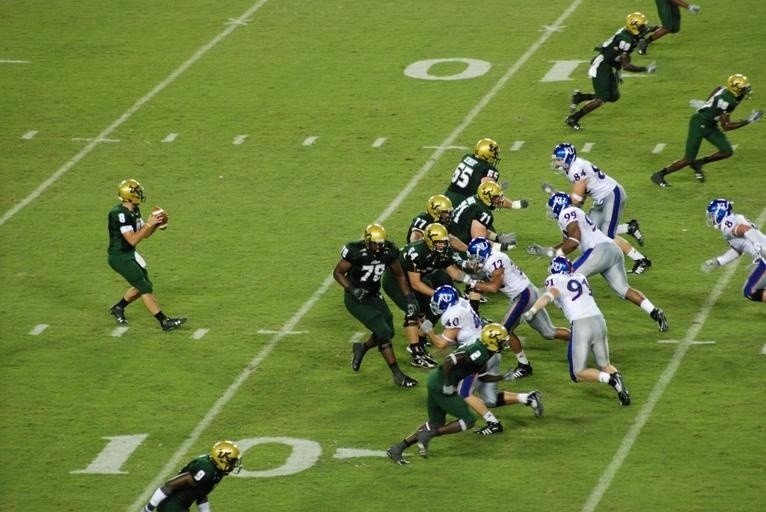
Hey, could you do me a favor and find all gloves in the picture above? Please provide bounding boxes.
[462,275,485,290]
[518,311,534,323]
[747,110,762,123]
[527,243,544,255]
[496,233,516,245]
[442,384,458,395]
[647,65,656,73]
[544,184,554,193]
[511,200,528,209]
[405,295,417,318]
[689,100,706,107]
[422,320,433,333]
[503,367,513,380]
[616,72,624,84]
[702,258,719,274]
[346,285,369,303]
[688,6,700,12]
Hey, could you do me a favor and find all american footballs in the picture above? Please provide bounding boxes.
[152,206,168,231]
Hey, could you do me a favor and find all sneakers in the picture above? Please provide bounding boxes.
[386,447,411,465]
[651,308,668,332]
[353,343,365,370]
[111,305,128,325]
[416,430,427,458]
[609,373,630,406]
[407,344,432,358]
[463,292,489,303]
[627,256,651,275]
[625,220,643,246]
[407,354,438,368]
[526,392,543,418]
[512,362,532,380]
[690,160,704,182]
[473,421,503,436]
[394,374,417,387]
[651,172,671,188]
[638,42,646,55]
[161,318,187,331]
[570,91,581,112]
[565,117,584,131]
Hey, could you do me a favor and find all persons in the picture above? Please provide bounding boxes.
[444,138,528,212]
[381,222,468,369]
[651,72,764,187]
[138,439,240,512]
[333,224,417,387]
[699,196,765,303]
[408,195,468,259]
[564,11,657,132]
[467,236,572,381]
[447,182,517,252]
[541,142,652,276]
[386,323,512,465]
[526,192,669,334]
[521,255,632,406]
[420,285,543,435]
[107,178,189,332]
[637,1,702,55]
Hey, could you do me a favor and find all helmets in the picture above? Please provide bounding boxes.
[430,285,459,315]
[118,180,146,206]
[211,441,243,473]
[547,191,571,220]
[427,195,455,225]
[363,224,386,254]
[549,257,573,275]
[728,74,752,101]
[466,237,492,270]
[478,181,504,209]
[475,138,500,168]
[425,223,449,255]
[481,323,510,353]
[626,12,649,38]
[706,199,733,229]
[551,144,576,176]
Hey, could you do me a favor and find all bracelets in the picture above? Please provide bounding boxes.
[144,223,152,230]
